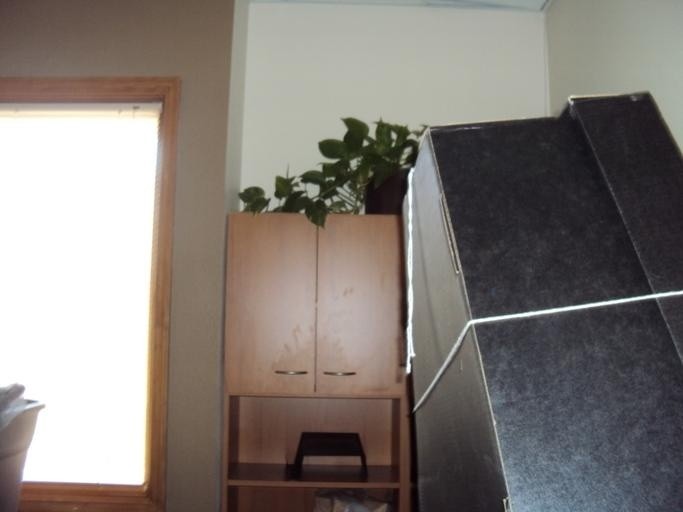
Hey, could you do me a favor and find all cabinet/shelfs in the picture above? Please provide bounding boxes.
[218,214,412,511]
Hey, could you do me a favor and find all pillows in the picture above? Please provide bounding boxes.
[0,383,25,409]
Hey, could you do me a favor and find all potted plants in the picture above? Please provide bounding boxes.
[238,116,417,228]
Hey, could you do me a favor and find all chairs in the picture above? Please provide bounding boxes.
[0,399,46,511]
